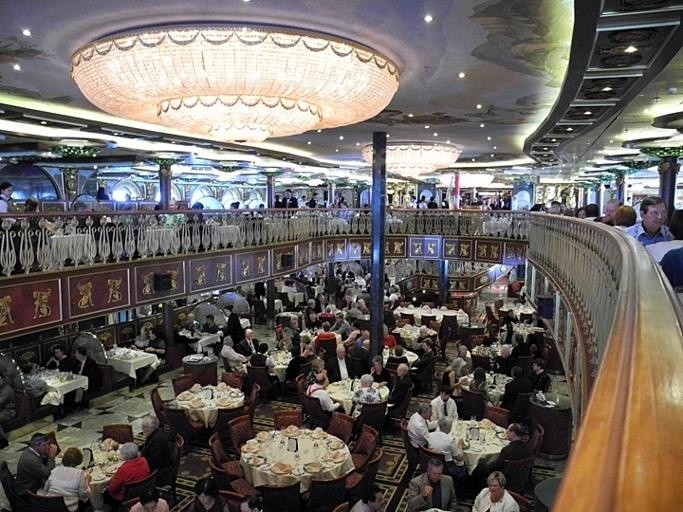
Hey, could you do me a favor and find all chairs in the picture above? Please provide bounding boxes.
[420,315,436,329]
[244,364,273,394]
[0,458,23,509]
[147,468,161,501]
[390,381,416,421]
[220,371,243,391]
[526,423,544,459]
[228,414,255,455]
[482,404,511,429]
[120,469,162,506]
[171,372,198,396]
[101,423,135,444]
[242,382,261,429]
[298,359,320,374]
[150,387,167,422]
[456,386,486,416]
[433,368,445,393]
[216,489,247,511]
[323,410,355,444]
[20,487,71,512]
[159,403,202,444]
[347,424,378,470]
[299,391,326,424]
[217,351,231,370]
[270,404,303,432]
[439,313,459,339]
[386,360,408,377]
[513,390,536,416]
[455,325,487,345]
[351,446,384,494]
[316,336,338,361]
[499,455,534,494]
[207,456,263,503]
[355,401,389,432]
[398,310,418,326]
[417,443,447,474]
[469,351,495,375]
[292,371,308,401]
[396,416,421,468]
[205,431,244,476]
[156,432,182,501]
[483,302,500,324]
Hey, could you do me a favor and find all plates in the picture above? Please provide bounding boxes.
[92,473,107,481]
[323,461,335,468]
[291,467,304,475]
[258,463,272,471]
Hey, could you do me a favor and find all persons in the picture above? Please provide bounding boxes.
[0,376,16,449]
[173,325,200,353]
[220,336,248,372]
[471,470,519,512]
[352,330,369,357]
[360,339,371,368]
[624,197,674,246]
[459,192,510,212]
[217,305,244,347]
[526,334,536,345]
[46,346,77,372]
[469,367,493,408]
[416,324,437,356]
[190,477,229,511]
[131,326,149,351]
[427,416,465,478]
[129,486,169,511]
[489,423,531,473]
[42,447,91,512]
[528,358,550,392]
[303,359,327,391]
[300,335,314,356]
[461,362,469,376]
[139,415,176,486]
[611,205,636,232]
[107,442,149,503]
[73,347,103,412]
[184,313,200,332]
[248,343,283,394]
[314,321,334,352]
[334,263,371,311]
[237,329,260,356]
[351,374,382,421]
[498,365,532,411]
[440,357,464,413]
[248,271,325,312]
[492,344,523,376]
[236,286,245,297]
[385,345,408,379]
[274,315,299,347]
[329,312,349,339]
[382,325,397,348]
[530,199,599,222]
[413,192,448,210]
[383,271,409,325]
[428,385,458,421]
[407,458,456,512]
[14,432,56,498]
[349,485,385,512]
[305,374,345,419]
[511,334,529,357]
[458,344,472,370]
[407,403,438,449]
[502,322,515,344]
[592,199,624,227]
[20,362,62,421]
[238,495,264,512]
[201,314,217,334]
[140,327,167,382]
[306,311,319,330]
[410,337,436,396]
[369,355,390,386]
[527,310,544,327]
[342,320,360,346]
[377,363,411,422]
[285,348,312,380]
[327,345,358,383]
[503,309,519,324]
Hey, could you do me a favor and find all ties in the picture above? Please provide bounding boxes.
[441,402,450,416]
[425,420,430,429]
[248,341,254,348]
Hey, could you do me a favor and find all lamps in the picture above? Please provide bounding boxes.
[440,171,494,188]
[360,140,464,185]
[67,23,402,149]
